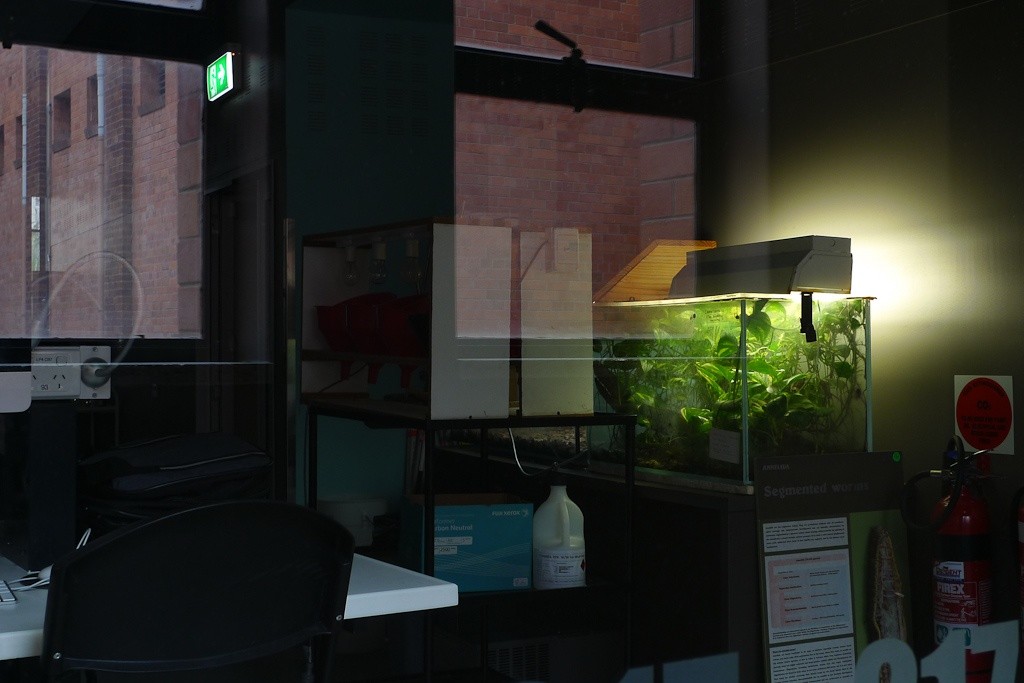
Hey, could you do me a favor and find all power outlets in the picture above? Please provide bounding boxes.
[30,347,112,399]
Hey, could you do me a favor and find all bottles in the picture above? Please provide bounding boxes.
[532,481,587,590]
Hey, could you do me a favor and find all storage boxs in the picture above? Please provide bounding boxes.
[432,292,878,495]
[665,236,853,297]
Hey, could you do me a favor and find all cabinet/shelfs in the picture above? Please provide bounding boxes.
[296,221,594,417]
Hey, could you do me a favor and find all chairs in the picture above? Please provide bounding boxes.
[40,433,356,682]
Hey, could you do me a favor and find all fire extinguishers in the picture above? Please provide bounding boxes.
[901,435,994,682]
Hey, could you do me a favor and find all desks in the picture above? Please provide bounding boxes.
[0,551,458,661]
